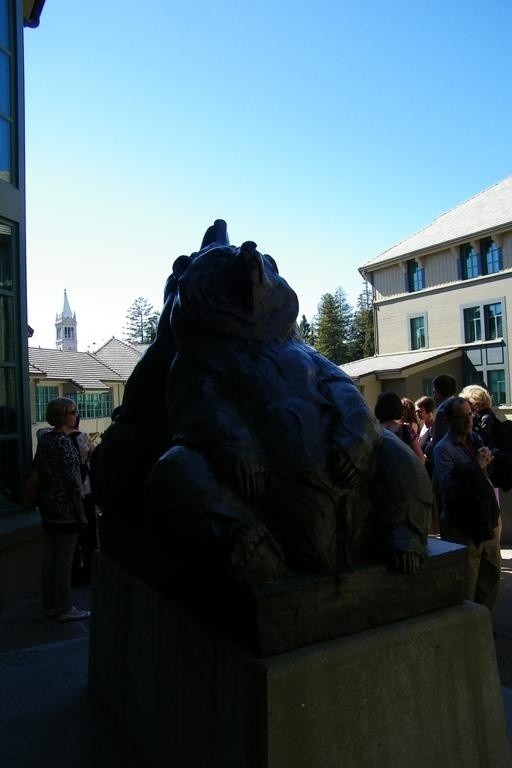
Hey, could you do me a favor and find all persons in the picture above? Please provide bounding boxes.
[32,396,93,622]
[414,394,438,444]
[396,397,420,424]
[67,411,93,590]
[423,371,491,481]
[432,396,503,614]
[457,381,505,521]
[372,389,427,466]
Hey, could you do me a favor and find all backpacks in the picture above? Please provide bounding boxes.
[482,419,511,492]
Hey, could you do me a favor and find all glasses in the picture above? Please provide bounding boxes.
[69,410,77,415]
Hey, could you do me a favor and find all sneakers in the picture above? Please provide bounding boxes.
[60,608,91,622]
